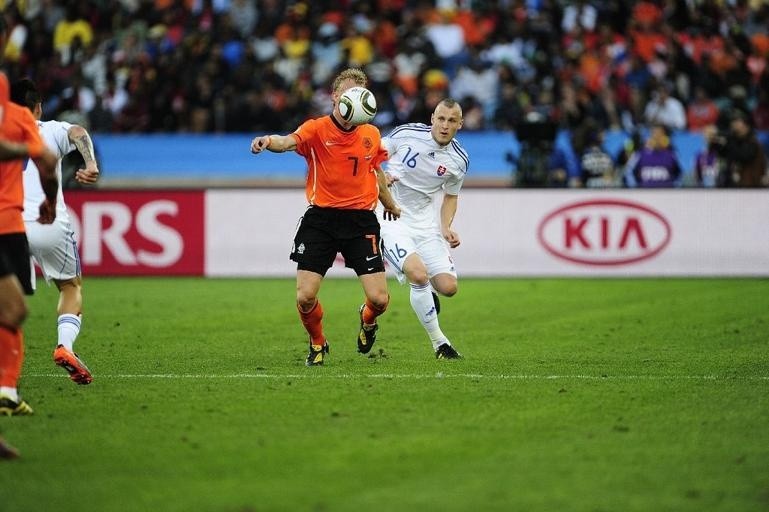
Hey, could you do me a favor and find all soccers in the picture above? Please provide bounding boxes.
[338,84,377,125]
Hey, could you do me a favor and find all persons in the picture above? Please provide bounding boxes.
[0,139,61,465]
[7,78,102,388]
[0,1,768,189]
[376,97,470,363]
[250,67,403,369]
[0,70,63,419]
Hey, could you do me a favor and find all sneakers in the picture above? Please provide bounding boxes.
[0,437,18,460]
[358,303,378,354]
[54,344,92,384]
[431,291,440,315]
[435,343,462,360]
[305,336,329,367]
[0,394,34,416]
